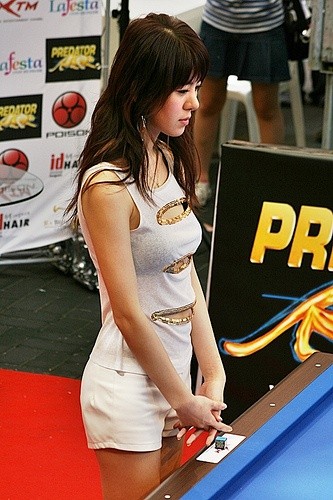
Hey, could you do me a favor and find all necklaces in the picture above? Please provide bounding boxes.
[149,167,160,188]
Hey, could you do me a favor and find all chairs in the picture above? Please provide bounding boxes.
[219,60,306,157]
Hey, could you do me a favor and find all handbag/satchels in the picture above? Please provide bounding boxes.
[282,0,312,61]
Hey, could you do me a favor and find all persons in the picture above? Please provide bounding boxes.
[189,0,292,206]
[62,13,233,500]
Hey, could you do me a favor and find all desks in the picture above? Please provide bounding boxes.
[141,352,333,500]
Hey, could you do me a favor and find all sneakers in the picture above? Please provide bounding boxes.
[187,181,212,206]
